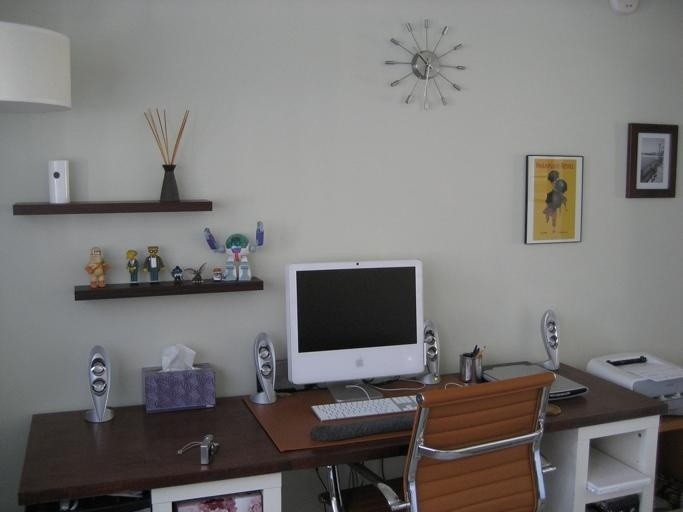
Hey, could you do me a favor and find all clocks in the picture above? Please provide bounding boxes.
[383,17,465,110]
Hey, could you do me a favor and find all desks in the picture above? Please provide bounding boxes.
[17,360,669,512]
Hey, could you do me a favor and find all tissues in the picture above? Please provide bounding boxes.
[143,338,218,413]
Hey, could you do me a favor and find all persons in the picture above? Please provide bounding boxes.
[123,249,141,285]
[201,219,264,282]
[143,245,164,284]
[170,263,184,282]
[84,245,110,289]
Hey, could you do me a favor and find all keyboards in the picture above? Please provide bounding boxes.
[311,394,424,423]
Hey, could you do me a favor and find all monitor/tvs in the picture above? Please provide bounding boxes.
[286,259,425,402]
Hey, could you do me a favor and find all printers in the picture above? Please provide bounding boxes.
[586,351,683,416]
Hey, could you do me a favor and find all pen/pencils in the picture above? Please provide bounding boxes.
[472,344,486,356]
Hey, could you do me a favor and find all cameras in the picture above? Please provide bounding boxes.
[200,434,219,465]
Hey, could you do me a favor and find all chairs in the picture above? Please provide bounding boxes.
[317,371,557,511]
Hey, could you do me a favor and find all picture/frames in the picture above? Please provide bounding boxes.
[524,154,584,245]
[625,122,678,199]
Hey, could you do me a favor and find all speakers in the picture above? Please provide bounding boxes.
[540,310,561,371]
[422,319,442,385]
[84,344,115,423]
[249,332,277,405]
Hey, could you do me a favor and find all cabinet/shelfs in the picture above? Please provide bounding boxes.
[12,196,265,302]
